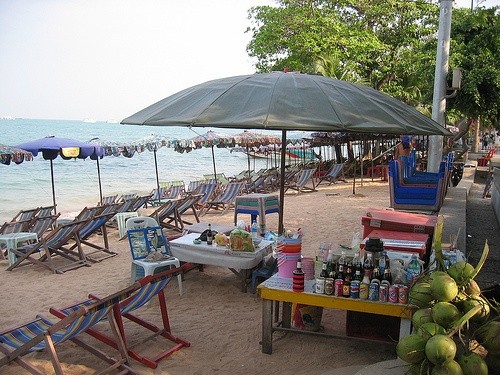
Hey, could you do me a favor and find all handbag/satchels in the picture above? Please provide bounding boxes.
[201,229,218,240]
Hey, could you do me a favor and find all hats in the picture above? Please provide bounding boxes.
[402,135,410,143]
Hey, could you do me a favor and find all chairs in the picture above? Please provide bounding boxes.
[0,162,347,375]
[479,149,495,166]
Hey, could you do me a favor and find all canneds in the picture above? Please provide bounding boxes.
[316,277,343,297]
[343,281,407,304]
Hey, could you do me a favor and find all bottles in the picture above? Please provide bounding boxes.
[314,250,391,284]
[430,250,457,274]
[292,260,305,292]
[251,220,257,238]
[406,255,420,283]
[206,224,213,246]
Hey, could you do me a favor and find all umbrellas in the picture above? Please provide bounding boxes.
[120,68,453,237]
[11,129,400,226]
[0,143,34,165]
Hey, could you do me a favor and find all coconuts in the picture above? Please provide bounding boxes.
[396,261,500,375]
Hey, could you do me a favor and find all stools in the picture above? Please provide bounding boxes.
[388,150,454,212]
[367,167,382,178]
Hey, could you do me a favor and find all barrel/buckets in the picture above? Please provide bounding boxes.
[291,303,323,332]
[300,258,315,280]
[277,237,301,279]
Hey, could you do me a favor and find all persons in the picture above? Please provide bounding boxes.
[396,135,412,159]
[482,132,500,150]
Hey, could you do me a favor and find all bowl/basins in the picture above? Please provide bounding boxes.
[252,238,262,246]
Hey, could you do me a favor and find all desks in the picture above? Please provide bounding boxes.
[0,233,42,268]
[168,233,274,292]
[110,211,138,238]
[256,273,414,358]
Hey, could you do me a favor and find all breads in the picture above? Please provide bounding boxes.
[214,232,253,251]
